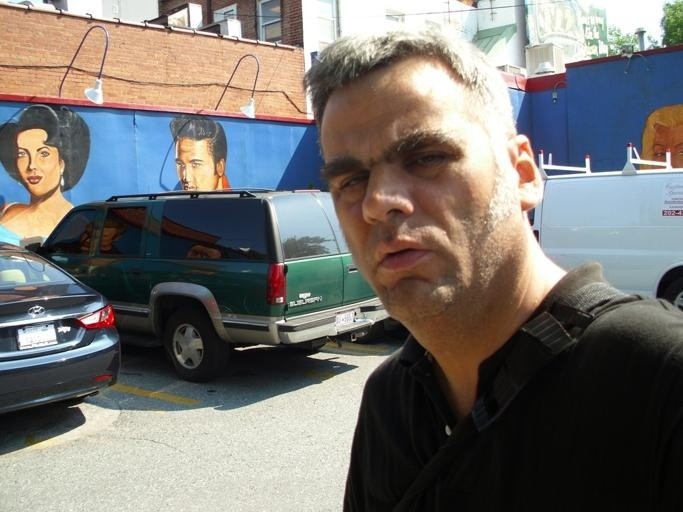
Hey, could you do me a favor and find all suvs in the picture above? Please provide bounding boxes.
[26,190,392,381]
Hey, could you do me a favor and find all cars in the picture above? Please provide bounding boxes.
[0,242,122,418]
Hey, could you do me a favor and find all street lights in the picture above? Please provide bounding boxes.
[635,28,645,52]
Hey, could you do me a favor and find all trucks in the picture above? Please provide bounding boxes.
[528,170,683,313]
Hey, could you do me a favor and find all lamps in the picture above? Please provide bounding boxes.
[213,55,260,120]
[59,23,109,106]
[551,81,566,104]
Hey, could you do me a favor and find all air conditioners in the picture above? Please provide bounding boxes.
[523,41,566,79]
[197,19,241,38]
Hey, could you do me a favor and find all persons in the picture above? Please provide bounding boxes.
[638,103,683,170]
[297,19,683,511]
[1,105,91,246]
[167,114,230,194]
